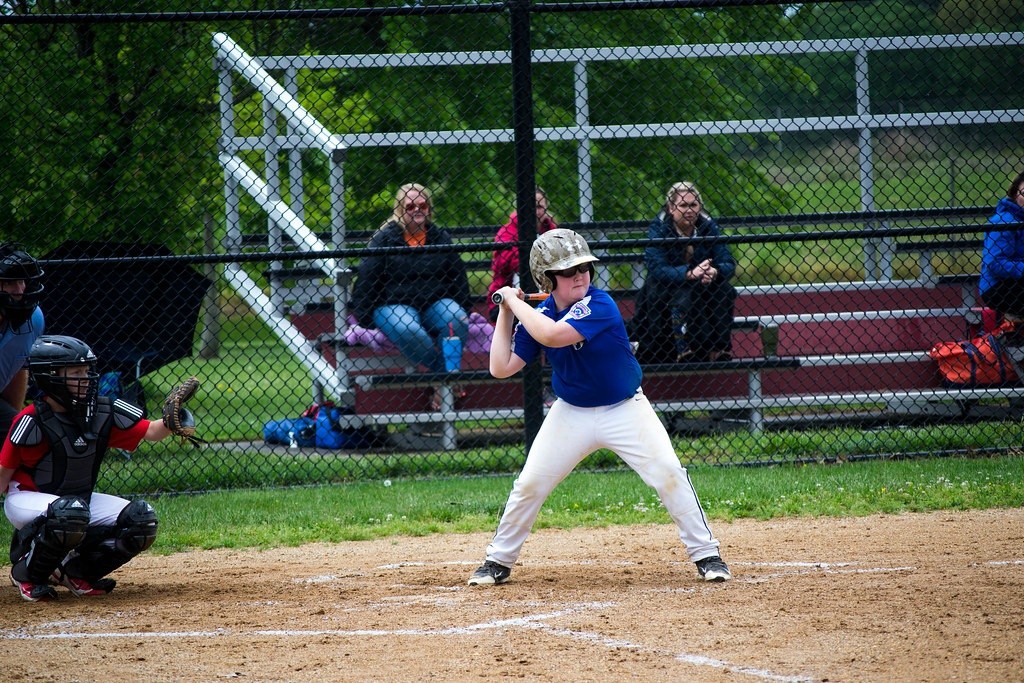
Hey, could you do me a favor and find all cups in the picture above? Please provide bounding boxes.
[761,324,779,359]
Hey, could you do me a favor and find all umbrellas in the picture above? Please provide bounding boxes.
[32,239,212,387]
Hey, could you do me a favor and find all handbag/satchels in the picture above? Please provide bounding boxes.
[264,401,390,449]
[931,321,1020,388]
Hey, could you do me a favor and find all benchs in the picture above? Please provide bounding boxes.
[241,203,1023,428]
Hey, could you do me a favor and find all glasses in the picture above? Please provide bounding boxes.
[549,262,592,278]
[405,202,427,211]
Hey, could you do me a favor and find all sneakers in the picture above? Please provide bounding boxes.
[696,556,731,581]
[53,568,107,598]
[467,561,511,586]
[8,574,49,602]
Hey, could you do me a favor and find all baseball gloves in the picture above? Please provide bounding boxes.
[162,376,200,437]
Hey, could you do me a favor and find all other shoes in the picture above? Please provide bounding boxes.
[712,352,732,361]
[677,351,694,363]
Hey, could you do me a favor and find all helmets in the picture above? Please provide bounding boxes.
[29,334,100,427]
[529,228,599,293]
[0,243,45,331]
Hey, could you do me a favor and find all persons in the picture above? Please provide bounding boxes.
[349,183,471,409]
[0,242,44,451]
[979,171,1024,362]
[468,227,730,585]
[0,335,200,602]
[638,181,737,367]
[487,185,559,334]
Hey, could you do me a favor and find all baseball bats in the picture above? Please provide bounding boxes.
[491,291,551,305]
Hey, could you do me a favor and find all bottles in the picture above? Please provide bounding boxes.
[443,337,462,373]
